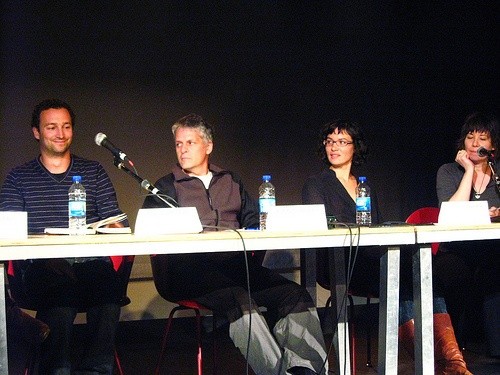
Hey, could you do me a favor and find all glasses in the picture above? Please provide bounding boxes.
[322,139,354,148]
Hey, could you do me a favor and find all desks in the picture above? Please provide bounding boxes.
[0,222,416,375]
[323,222,500,375]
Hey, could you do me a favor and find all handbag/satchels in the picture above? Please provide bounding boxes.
[5,285,50,375]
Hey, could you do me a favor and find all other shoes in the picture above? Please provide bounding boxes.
[285,366,318,375]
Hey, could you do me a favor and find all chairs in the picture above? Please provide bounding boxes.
[4,207,439,375]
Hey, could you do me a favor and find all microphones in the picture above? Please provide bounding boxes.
[477,147,500,158]
[94,132,125,160]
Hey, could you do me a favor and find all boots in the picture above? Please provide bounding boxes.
[434,313,474,375]
[399,317,444,375]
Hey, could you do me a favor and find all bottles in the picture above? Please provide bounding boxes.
[258,175,276,231]
[68,176,86,236]
[355,177,371,226]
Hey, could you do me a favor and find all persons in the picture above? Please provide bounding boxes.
[436,112,500,356]
[301,123,472,375]
[143,116,330,375]
[0,100,129,375]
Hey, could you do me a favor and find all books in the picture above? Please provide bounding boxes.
[46,212,131,235]
